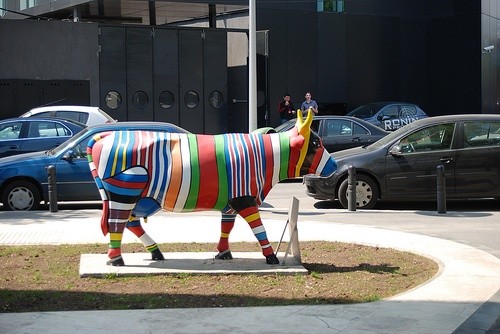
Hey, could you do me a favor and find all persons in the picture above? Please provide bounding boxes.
[311,121,317,133]
[301,91,318,116]
[278,94,296,124]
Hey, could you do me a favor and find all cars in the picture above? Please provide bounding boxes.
[346,101,431,132]
[275,115,392,169]
[0,122,196,212]
[16,105,115,128]
[0,116,91,157]
[304,113,500,207]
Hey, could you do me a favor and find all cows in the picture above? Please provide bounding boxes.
[85,109,338,267]
